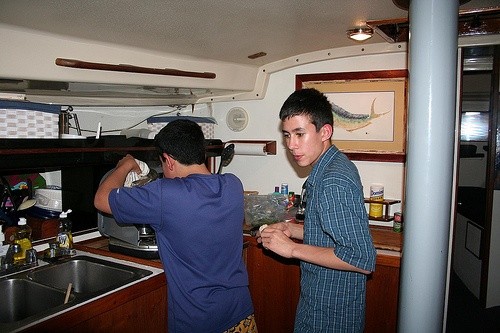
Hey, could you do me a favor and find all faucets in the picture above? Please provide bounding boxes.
[0,243,48,279]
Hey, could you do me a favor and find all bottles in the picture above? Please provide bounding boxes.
[272,183,303,208]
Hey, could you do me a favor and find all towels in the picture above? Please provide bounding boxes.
[124,159,150,188]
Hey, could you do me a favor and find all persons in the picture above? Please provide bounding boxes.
[94,120,256,333]
[257,89,376,333]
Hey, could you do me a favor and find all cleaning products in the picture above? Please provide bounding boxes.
[11,217,33,264]
[55,208,74,256]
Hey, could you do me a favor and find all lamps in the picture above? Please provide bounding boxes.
[347,28,374,41]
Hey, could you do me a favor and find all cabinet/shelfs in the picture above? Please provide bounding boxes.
[60,242,400,333]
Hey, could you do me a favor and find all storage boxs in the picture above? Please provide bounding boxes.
[0,100,61,138]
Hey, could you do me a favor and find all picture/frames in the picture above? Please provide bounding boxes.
[295,70,407,164]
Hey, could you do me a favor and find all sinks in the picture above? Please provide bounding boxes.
[19,254,154,299]
[0,273,82,333]
